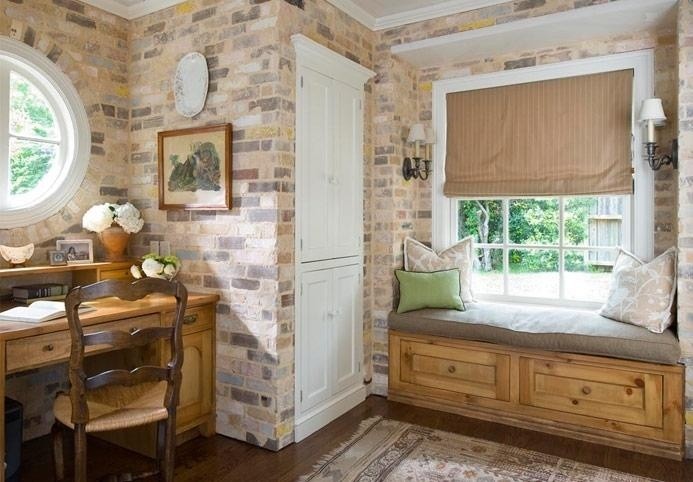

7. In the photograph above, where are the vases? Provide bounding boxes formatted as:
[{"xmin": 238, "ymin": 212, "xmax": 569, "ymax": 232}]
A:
[{"xmin": 99, "ymin": 223, "xmax": 129, "ymax": 262}]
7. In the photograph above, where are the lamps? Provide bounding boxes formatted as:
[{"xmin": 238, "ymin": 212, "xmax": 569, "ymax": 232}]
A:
[
  {"xmin": 403, "ymin": 122, "xmax": 435, "ymax": 181},
  {"xmin": 637, "ymin": 98, "xmax": 679, "ymax": 171}
]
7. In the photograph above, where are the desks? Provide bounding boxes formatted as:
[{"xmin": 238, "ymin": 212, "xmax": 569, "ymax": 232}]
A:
[{"xmin": 0, "ymin": 261, "xmax": 220, "ymax": 482}]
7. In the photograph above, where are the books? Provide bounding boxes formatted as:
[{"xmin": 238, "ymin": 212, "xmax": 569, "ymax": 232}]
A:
[
  {"xmin": 0, "ymin": 301, "xmax": 97, "ymax": 324},
  {"xmin": 11, "ymin": 283, "xmax": 68, "ymax": 299},
  {"xmin": 13, "ymin": 295, "xmax": 67, "ymax": 304}
]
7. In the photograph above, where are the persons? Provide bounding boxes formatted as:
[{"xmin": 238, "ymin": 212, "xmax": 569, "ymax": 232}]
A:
[{"xmin": 66, "ymin": 247, "xmax": 78, "ymax": 261}]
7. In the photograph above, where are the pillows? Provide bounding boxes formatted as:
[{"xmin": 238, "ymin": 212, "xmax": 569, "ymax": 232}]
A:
[
  {"xmin": 598, "ymin": 246, "xmax": 679, "ymax": 334},
  {"xmin": 393, "ymin": 268, "xmax": 466, "ymax": 314},
  {"xmin": 404, "ymin": 236, "xmax": 473, "ymax": 302}
]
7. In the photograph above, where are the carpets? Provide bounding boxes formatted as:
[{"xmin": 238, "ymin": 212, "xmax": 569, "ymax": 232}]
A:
[{"xmin": 296, "ymin": 413, "xmax": 662, "ymax": 482}]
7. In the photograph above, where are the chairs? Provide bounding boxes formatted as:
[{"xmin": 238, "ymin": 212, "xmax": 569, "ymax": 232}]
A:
[{"xmin": 49, "ymin": 277, "xmax": 189, "ymax": 482}]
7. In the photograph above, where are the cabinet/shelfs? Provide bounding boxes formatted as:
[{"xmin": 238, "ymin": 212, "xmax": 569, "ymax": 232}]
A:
[{"xmin": 386, "ymin": 296, "xmax": 683, "ymax": 461}]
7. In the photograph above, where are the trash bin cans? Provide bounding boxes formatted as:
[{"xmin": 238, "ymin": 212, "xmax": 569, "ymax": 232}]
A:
[{"xmin": 4, "ymin": 396, "xmax": 23, "ymax": 481}]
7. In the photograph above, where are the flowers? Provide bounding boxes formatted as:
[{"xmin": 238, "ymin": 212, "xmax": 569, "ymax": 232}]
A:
[
  {"xmin": 82, "ymin": 202, "xmax": 144, "ymax": 234},
  {"xmin": 131, "ymin": 253, "xmax": 181, "ymax": 282}
]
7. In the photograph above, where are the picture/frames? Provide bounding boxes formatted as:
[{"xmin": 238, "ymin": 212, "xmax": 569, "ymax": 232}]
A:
[
  {"xmin": 158, "ymin": 123, "xmax": 230, "ymax": 211},
  {"xmin": 50, "ymin": 250, "xmax": 68, "ymax": 266},
  {"xmin": 57, "ymin": 239, "xmax": 94, "ymax": 264}
]
